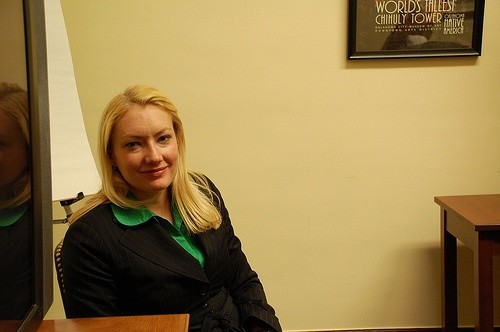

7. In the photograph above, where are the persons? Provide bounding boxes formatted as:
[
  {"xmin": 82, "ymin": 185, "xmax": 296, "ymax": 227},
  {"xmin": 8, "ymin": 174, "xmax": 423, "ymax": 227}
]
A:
[
  {"xmin": 61, "ymin": 85, "xmax": 282, "ymax": 332},
  {"xmin": 0, "ymin": 81, "xmax": 35, "ymax": 316}
]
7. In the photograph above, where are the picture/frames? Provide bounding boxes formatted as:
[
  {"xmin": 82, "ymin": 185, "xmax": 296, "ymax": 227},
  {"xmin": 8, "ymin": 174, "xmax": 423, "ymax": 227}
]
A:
[{"xmin": 346, "ymin": 0, "xmax": 486, "ymax": 61}]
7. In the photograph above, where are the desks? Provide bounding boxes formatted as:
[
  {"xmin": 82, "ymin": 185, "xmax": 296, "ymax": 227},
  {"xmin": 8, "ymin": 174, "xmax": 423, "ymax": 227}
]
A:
[
  {"xmin": 435, "ymin": 194, "xmax": 500, "ymax": 332},
  {"xmin": 37, "ymin": 313, "xmax": 191, "ymax": 332}
]
